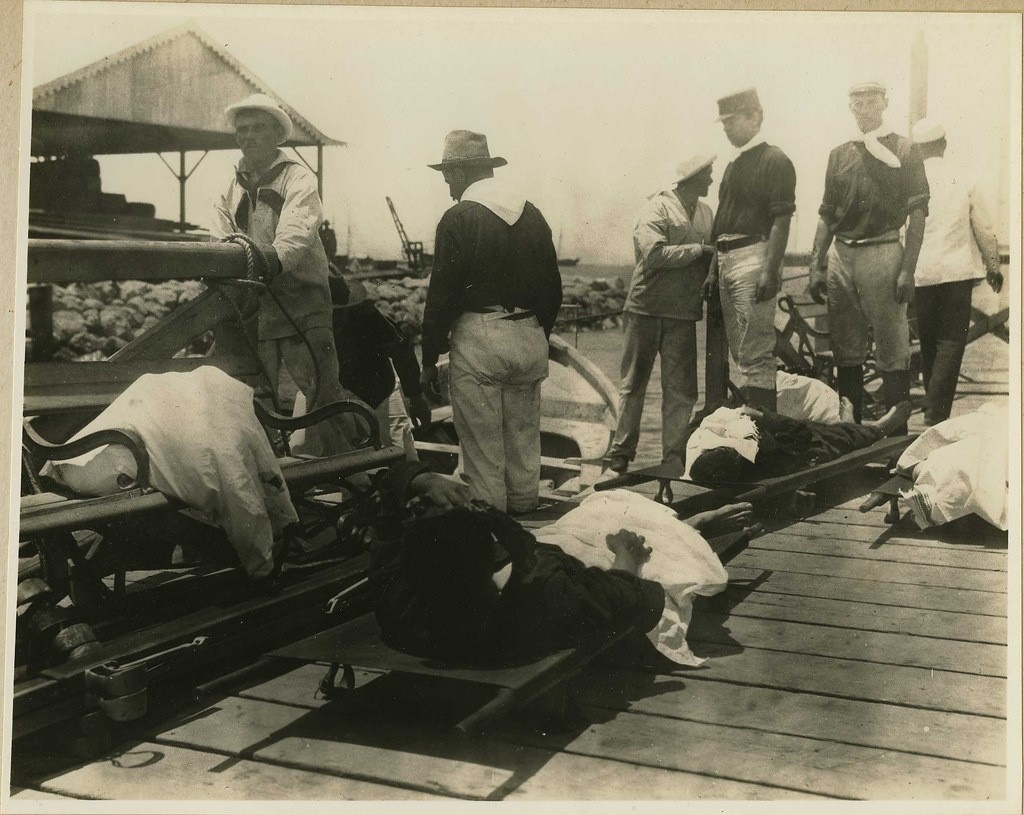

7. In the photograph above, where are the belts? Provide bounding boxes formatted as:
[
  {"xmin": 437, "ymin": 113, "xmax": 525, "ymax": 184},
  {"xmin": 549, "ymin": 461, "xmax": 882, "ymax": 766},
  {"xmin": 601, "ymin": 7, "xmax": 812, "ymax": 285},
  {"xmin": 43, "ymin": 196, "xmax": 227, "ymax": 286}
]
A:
[
  {"xmin": 834, "ymin": 238, "xmax": 900, "ymax": 247},
  {"xmin": 716, "ymin": 234, "xmax": 762, "ymax": 253},
  {"xmin": 465, "ymin": 307, "xmax": 536, "ymax": 320}
]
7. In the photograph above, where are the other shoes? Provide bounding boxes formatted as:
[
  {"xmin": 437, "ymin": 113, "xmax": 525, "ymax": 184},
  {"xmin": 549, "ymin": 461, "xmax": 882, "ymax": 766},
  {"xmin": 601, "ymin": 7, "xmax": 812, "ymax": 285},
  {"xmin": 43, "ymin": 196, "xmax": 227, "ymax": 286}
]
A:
[{"xmin": 609, "ymin": 457, "xmax": 629, "ymax": 472}]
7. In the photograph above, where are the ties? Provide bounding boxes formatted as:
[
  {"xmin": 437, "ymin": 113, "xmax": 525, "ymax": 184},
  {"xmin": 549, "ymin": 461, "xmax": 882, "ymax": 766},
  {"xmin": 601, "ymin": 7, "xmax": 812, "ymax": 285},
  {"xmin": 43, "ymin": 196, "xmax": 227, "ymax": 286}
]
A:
[
  {"xmin": 460, "ymin": 178, "xmax": 527, "ymax": 226},
  {"xmin": 237, "ymin": 161, "xmax": 287, "ymax": 212},
  {"xmin": 729, "ymin": 132, "xmax": 781, "ymax": 164},
  {"xmin": 838, "ymin": 123, "xmax": 901, "ymax": 168}
]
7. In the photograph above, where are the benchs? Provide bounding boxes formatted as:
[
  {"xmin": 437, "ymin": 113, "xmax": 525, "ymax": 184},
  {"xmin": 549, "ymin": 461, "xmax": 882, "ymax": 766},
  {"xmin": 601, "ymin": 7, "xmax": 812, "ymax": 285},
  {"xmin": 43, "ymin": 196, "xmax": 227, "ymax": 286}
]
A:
[
  {"xmin": 19, "ymin": 358, "xmax": 405, "ymax": 623},
  {"xmin": 775, "ymin": 290, "xmax": 923, "ymax": 414}
]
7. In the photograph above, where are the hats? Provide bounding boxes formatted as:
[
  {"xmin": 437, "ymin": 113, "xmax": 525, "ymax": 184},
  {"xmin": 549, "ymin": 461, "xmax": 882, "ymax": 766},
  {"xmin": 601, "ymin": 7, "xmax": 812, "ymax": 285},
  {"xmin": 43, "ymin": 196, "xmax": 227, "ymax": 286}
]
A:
[
  {"xmin": 670, "ymin": 144, "xmax": 717, "ymax": 185},
  {"xmin": 427, "ymin": 129, "xmax": 507, "ymax": 171},
  {"xmin": 714, "ymin": 86, "xmax": 761, "ymax": 123},
  {"xmin": 848, "ymin": 72, "xmax": 886, "ymax": 96},
  {"xmin": 911, "ymin": 118, "xmax": 944, "ymax": 144},
  {"xmin": 225, "ymin": 94, "xmax": 293, "ymax": 145}
]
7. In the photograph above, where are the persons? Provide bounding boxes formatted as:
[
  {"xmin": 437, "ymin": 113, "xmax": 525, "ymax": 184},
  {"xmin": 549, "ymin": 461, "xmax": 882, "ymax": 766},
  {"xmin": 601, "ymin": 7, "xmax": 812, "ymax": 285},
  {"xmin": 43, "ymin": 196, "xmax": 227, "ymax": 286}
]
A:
[
  {"xmin": 420, "ymin": 130, "xmax": 564, "ymax": 513},
  {"xmin": 677, "ymin": 402, "xmax": 912, "ymax": 486},
  {"xmin": 211, "ymin": 95, "xmax": 381, "ymax": 513},
  {"xmin": 810, "ymin": 75, "xmax": 930, "ymax": 434},
  {"xmin": 292, "ymin": 262, "xmax": 431, "ymax": 461},
  {"xmin": 900, "ymin": 118, "xmax": 1004, "ymax": 425},
  {"xmin": 608, "ymin": 153, "xmax": 715, "ymax": 479},
  {"xmin": 378, "ymin": 461, "xmax": 664, "ymax": 658},
  {"xmin": 703, "ymin": 88, "xmax": 796, "ymax": 413}
]
